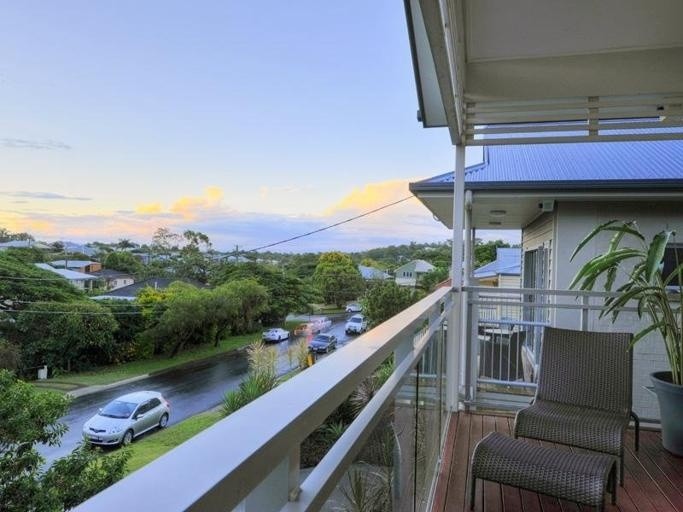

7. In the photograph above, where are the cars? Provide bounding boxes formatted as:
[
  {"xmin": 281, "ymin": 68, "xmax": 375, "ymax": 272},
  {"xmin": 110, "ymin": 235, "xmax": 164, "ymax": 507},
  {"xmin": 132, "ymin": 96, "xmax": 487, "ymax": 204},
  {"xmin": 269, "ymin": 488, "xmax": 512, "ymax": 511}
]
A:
[
  {"xmin": 293, "ymin": 322, "xmax": 319, "ymax": 338},
  {"xmin": 261, "ymin": 327, "xmax": 291, "ymax": 342},
  {"xmin": 344, "ymin": 302, "xmax": 362, "ymax": 313},
  {"xmin": 311, "ymin": 316, "xmax": 332, "ymax": 331},
  {"xmin": 79, "ymin": 389, "xmax": 171, "ymax": 450},
  {"xmin": 306, "ymin": 333, "xmax": 338, "ymax": 356}
]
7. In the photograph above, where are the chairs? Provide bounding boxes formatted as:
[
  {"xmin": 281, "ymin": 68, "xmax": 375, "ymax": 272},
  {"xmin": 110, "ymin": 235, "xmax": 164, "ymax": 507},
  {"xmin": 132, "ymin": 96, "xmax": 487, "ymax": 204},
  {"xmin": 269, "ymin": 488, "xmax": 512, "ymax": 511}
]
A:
[{"xmin": 466, "ymin": 329, "xmax": 637, "ymax": 511}]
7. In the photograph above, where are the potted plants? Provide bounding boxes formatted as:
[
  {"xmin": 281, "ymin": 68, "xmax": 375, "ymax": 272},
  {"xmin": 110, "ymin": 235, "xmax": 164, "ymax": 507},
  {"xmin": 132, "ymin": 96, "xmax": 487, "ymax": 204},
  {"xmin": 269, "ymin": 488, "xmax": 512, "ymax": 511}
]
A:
[{"xmin": 566, "ymin": 217, "xmax": 683, "ymax": 458}]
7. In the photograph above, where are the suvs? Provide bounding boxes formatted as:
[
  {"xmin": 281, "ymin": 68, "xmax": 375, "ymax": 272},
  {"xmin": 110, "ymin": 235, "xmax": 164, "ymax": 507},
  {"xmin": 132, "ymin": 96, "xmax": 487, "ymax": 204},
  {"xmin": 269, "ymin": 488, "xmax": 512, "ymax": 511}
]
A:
[{"xmin": 343, "ymin": 314, "xmax": 367, "ymax": 336}]
